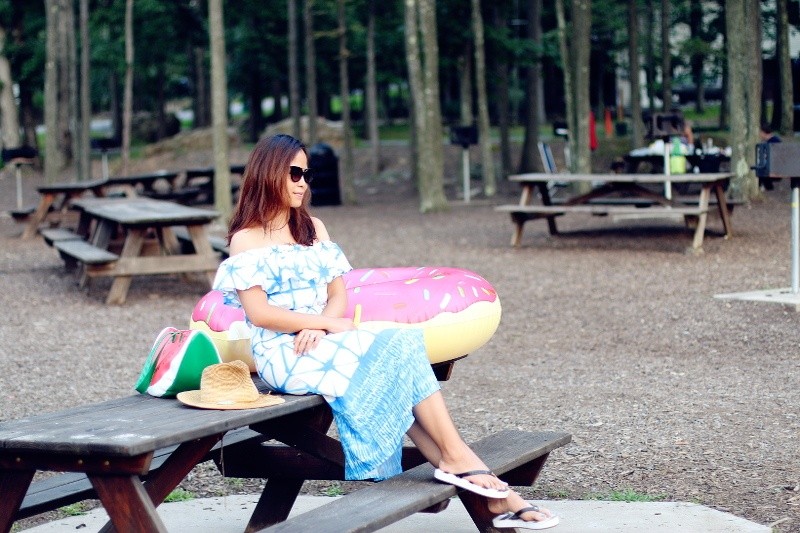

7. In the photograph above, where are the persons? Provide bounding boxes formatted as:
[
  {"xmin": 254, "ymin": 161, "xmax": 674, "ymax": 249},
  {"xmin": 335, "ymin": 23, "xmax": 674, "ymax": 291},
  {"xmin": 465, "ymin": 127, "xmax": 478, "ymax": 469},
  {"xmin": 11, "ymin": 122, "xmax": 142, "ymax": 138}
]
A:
[
  {"xmin": 212, "ymin": 134, "xmax": 561, "ymax": 530},
  {"xmin": 665, "ymin": 108, "xmax": 782, "ymax": 228}
]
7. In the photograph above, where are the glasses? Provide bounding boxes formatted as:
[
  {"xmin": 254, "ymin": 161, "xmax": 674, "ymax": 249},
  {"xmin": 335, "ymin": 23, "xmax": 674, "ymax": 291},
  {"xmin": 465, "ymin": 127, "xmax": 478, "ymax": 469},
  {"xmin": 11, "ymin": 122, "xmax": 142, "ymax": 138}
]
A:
[{"xmin": 289, "ymin": 166, "xmax": 314, "ymax": 184}]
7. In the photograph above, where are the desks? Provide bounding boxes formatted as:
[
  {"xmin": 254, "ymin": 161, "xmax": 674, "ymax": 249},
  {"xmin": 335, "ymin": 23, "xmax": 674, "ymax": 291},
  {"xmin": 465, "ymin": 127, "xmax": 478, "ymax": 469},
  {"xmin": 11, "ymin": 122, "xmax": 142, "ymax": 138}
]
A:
[
  {"xmin": 626, "ymin": 151, "xmax": 729, "ymax": 190},
  {"xmin": 113, "ymin": 171, "xmax": 181, "ymax": 198},
  {"xmin": 25, "ymin": 176, "xmax": 109, "ymax": 240},
  {"xmin": 509, "ymin": 172, "xmax": 733, "ymax": 249},
  {"xmin": 181, "ymin": 164, "xmax": 251, "ymax": 204},
  {"xmin": 67, "ymin": 196, "xmax": 220, "ymax": 307},
  {"xmin": 1, "ymin": 354, "xmax": 466, "ymax": 533}
]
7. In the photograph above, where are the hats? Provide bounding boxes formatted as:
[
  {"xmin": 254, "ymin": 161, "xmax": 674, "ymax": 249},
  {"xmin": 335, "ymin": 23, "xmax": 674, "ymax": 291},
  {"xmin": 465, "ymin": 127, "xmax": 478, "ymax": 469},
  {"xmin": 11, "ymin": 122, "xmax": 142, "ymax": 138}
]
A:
[{"xmin": 176, "ymin": 359, "xmax": 284, "ymax": 410}]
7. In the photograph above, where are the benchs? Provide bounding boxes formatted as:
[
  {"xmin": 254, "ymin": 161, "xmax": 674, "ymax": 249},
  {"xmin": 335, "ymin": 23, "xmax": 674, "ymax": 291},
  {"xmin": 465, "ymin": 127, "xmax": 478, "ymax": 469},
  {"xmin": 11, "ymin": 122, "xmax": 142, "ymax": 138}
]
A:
[
  {"xmin": 502, "ymin": 195, "xmax": 746, "ymax": 252},
  {"xmin": 1, "ymin": 428, "xmax": 572, "ymax": 533},
  {"xmin": 11, "ymin": 184, "xmax": 242, "ymax": 307}
]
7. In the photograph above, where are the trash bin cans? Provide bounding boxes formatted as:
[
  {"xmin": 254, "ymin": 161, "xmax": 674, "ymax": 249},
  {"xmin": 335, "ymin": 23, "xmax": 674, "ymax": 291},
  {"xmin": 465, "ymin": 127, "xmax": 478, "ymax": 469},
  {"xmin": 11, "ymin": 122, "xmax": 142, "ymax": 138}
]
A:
[{"xmin": 308, "ymin": 143, "xmax": 342, "ymax": 206}]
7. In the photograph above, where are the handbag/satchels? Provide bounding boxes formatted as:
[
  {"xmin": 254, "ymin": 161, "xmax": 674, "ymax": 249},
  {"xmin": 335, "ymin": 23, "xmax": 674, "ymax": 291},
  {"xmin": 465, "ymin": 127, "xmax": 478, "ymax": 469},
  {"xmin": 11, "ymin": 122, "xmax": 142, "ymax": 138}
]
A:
[{"xmin": 135, "ymin": 326, "xmax": 223, "ymax": 398}]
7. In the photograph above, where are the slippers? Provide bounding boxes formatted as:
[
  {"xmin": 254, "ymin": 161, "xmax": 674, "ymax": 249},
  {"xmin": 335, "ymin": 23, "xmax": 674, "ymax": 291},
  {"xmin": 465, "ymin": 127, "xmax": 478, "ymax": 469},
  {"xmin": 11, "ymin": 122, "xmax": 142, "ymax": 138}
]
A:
[
  {"xmin": 434, "ymin": 469, "xmax": 509, "ymax": 499},
  {"xmin": 493, "ymin": 503, "xmax": 559, "ymax": 530}
]
